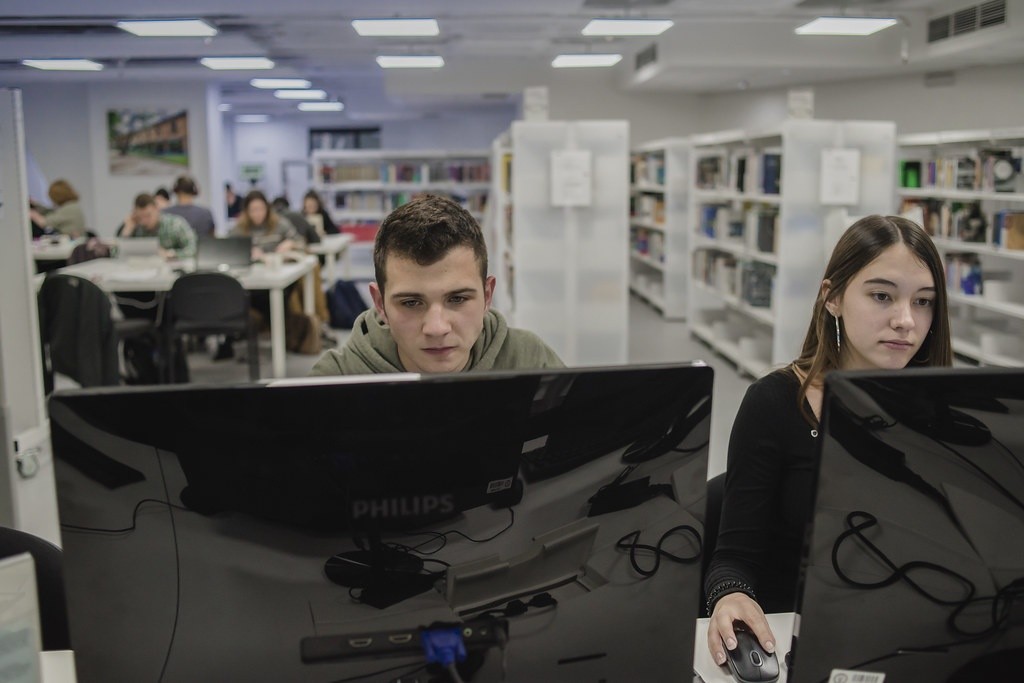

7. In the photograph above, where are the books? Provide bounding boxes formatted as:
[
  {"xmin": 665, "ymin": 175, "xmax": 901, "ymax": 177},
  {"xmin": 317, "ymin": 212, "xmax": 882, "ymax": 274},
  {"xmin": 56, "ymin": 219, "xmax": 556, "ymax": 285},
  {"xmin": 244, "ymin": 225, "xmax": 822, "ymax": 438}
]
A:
[
  {"xmin": 629, "ymin": 152, "xmax": 783, "ymax": 307},
  {"xmin": 321, "ymin": 156, "xmax": 521, "ymax": 297},
  {"xmin": 899, "ymin": 146, "xmax": 1024, "ymax": 294}
]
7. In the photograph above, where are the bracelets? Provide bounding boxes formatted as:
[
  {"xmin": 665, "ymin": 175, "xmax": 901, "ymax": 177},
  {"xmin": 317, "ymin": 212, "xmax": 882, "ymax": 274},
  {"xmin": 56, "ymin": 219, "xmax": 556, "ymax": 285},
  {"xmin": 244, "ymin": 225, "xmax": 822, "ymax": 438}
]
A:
[{"xmin": 706, "ymin": 580, "xmax": 757, "ymax": 616}]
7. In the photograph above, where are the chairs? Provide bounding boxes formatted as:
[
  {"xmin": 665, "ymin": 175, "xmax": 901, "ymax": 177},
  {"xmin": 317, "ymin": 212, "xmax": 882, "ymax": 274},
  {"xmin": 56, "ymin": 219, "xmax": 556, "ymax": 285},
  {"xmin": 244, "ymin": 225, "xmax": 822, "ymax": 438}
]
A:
[
  {"xmin": 0, "ymin": 527, "xmax": 72, "ymax": 650},
  {"xmin": 42, "ymin": 266, "xmax": 260, "ymax": 382}
]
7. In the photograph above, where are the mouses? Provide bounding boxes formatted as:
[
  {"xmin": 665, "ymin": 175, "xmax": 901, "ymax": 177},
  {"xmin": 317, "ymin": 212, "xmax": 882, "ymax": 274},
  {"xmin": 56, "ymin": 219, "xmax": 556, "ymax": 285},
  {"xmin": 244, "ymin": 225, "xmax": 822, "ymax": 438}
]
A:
[{"xmin": 722, "ymin": 630, "xmax": 780, "ymax": 683}]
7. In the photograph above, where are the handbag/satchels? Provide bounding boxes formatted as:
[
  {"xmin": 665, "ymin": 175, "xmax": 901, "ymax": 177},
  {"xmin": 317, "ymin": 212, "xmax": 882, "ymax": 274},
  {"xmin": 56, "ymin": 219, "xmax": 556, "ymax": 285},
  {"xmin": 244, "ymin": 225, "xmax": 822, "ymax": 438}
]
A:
[{"xmin": 285, "ymin": 314, "xmax": 320, "ymax": 353}]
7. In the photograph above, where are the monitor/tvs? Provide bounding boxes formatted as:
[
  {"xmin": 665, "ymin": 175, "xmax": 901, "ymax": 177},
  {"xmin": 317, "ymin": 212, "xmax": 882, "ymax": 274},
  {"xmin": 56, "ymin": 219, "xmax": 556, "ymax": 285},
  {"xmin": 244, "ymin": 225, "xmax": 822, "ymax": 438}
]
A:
[
  {"xmin": 789, "ymin": 365, "xmax": 1024, "ymax": 683},
  {"xmin": 48, "ymin": 357, "xmax": 716, "ymax": 683}
]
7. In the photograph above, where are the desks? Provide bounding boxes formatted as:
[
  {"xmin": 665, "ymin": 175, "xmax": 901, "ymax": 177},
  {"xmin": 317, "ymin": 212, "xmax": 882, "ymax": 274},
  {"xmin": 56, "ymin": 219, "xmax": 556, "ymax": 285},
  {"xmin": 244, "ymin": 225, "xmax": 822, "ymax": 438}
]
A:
[
  {"xmin": 35, "ymin": 252, "xmax": 316, "ymax": 384},
  {"xmin": 42, "ymin": 611, "xmax": 796, "ymax": 683}
]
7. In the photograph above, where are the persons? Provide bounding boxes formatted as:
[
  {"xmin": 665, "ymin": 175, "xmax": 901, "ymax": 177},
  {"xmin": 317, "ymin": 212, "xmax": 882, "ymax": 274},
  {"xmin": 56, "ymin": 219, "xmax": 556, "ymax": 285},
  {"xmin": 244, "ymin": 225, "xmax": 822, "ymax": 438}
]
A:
[
  {"xmin": 303, "ymin": 193, "xmax": 570, "ymax": 377},
  {"xmin": 27, "ymin": 180, "xmax": 87, "ymax": 257},
  {"xmin": 113, "ymin": 175, "xmax": 340, "ymax": 343},
  {"xmin": 704, "ymin": 215, "xmax": 956, "ymax": 667}
]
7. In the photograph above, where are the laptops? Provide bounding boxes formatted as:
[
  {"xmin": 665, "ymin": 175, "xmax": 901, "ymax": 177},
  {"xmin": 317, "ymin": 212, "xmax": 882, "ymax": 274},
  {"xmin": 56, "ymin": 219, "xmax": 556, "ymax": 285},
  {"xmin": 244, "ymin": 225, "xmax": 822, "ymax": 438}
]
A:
[
  {"xmin": 117, "ymin": 236, "xmax": 160, "ymax": 262},
  {"xmin": 194, "ymin": 236, "xmax": 254, "ymax": 281}
]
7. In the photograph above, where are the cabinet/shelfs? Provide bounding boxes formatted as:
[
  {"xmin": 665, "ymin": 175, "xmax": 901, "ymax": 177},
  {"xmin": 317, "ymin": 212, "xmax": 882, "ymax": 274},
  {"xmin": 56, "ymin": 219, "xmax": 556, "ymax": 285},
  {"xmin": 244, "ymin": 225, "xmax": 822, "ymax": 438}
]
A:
[
  {"xmin": 902, "ymin": 127, "xmax": 1024, "ymax": 371},
  {"xmin": 486, "ymin": 117, "xmax": 632, "ymax": 366},
  {"xmin": 632, "ymin": 137, "xmax": 693, "ymax": 321},
  {"xmin": 195, "ymin": 225, "xmax": 358, "ymax": 278},
  {"xmin": 688, "ymin": 119, "xmax": 902, "ymax": 381},
  {"xmin": 313, "ymin": 148, "xmax": 486, "ymax": 270}
]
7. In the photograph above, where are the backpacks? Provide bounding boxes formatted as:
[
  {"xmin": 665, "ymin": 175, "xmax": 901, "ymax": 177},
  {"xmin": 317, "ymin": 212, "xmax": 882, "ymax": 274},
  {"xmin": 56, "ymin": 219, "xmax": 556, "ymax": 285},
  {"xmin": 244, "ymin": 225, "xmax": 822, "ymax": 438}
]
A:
[{"xmin": 326, "ymin": 279, "xmax": 367, "ymax": 329}]
